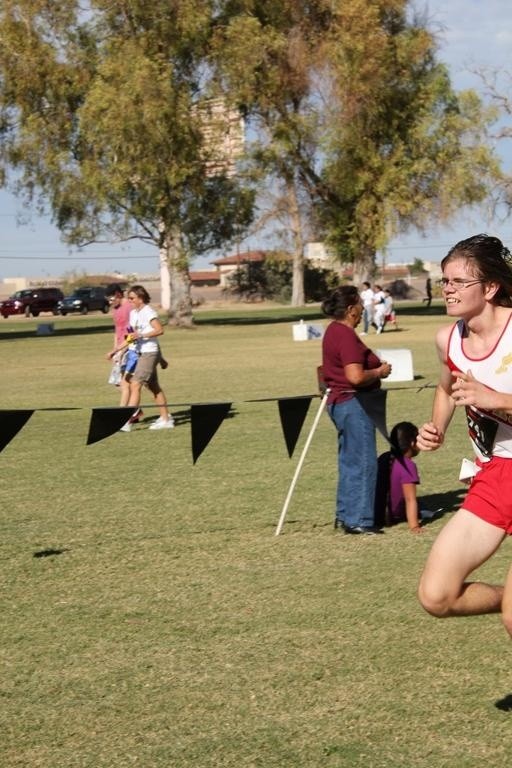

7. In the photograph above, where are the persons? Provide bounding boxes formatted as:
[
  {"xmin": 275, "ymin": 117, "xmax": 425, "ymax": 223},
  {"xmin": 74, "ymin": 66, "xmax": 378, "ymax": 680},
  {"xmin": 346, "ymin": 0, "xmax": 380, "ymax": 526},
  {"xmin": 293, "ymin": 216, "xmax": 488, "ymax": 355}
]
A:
[
  {"xmin": 422, "ymin": 278, "xmax": 432, "ymax": 307},
  {"xmin": 322, "ymin": 286, "xmax": 438, "ymax": 534},
  {"xmin": 103, "ymin": 283, "xmax": 175, "ymax": 432},
  {"xmin": 360, "ymin": 282, "xmax": 397, "ymax": 335},
  {"xmin": 416, "ymin": 234, "xmax": 512, "ymax": 639}
]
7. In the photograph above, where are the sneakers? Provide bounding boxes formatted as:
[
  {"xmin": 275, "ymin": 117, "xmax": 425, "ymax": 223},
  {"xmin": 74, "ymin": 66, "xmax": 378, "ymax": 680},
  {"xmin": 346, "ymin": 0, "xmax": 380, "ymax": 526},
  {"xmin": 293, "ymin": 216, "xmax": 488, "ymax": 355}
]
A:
[
  {"xmin": 148, "ymin": 419, "xmax": 173, "ymax": 430},
  {"xmin": 128, "ymin": 408, "xmax": 144, "ymax": 424},
  {"xmin": 121, "ymin": 422, "xmax": 134, "ymax": 433}
]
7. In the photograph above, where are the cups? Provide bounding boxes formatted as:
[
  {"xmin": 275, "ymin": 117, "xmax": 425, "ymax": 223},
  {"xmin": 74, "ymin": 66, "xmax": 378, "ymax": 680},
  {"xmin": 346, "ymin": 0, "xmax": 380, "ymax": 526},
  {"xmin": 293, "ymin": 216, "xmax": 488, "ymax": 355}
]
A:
[{"xmin": 126, "ymin": 324, "xmax": 138, "ymax": 345}]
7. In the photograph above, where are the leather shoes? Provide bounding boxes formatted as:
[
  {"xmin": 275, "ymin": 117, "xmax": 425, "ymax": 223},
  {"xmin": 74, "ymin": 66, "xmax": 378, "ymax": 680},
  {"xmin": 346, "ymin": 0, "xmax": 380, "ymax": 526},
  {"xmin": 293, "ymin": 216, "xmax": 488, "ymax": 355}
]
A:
[{"xmin": 334, "ymin": 518, "xmax": 385, "ymax": 535}]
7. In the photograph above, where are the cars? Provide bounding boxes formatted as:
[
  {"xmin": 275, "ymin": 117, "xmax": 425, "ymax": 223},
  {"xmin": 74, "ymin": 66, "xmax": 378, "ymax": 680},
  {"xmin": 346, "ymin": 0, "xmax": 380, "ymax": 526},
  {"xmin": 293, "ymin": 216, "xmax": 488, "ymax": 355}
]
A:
[{"xmin": 0, "ymin": 286, "xmax": 110, "ymax": 318}]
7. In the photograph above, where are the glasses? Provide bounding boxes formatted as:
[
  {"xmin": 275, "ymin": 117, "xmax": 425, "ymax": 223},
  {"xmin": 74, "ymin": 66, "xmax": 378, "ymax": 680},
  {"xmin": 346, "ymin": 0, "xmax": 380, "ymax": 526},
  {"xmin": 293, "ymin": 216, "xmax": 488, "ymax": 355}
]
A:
[
  {"xmin": 128, "ymin": 297, "xmax": 135, "ymax": 301},
  {"xmin": 436, "ymin": 278, "xmax": 489, "ymax": 290}
]
